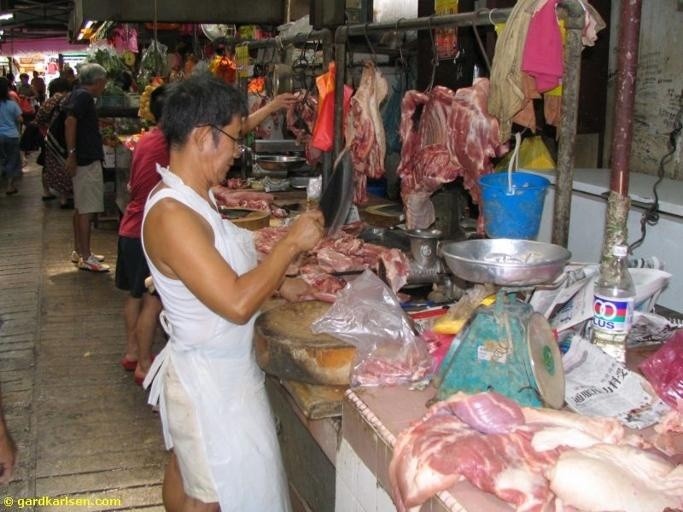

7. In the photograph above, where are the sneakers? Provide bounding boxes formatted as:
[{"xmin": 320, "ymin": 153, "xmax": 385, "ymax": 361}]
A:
[
  {"xmin": 42, "ymin": 194, "xmax": 56, "ymax": 200},
  {"xmin": 78, "ymin": 253, "xmax": 110, "ymax": 272},
  {"xmin": 6, "ymin": 188, "xmax": 18, "ymax": 195},
  {"xmin": 61, "ymin": 199, "xmax": 74, "ymax": 209},
  {"xmin": 72, "ymin": 250, "xmax": 105, "ymax": 262}
]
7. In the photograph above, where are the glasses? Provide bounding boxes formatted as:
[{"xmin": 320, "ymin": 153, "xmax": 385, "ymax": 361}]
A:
[{"xmin": 196, "ymin": 124, "xmax": 244, "ymax": 147}]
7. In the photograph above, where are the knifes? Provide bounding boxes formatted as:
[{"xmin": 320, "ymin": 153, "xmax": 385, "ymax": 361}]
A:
[{"xmin": 315, "ymin": 149, "xmax": 353, "ymax": 231}]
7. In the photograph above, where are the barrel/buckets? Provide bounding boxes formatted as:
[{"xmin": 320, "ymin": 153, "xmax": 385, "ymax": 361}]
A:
[{"xmin": 475, "ymin": 131, "xmax": 551, "ymax": 240}]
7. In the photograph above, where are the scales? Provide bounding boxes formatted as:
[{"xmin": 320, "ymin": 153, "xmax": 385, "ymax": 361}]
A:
[
  {"xmin": 425, "ymin": 237, "xmax": 571, "ymax": 409},
  {"xmin": 255, "ymin": 36, "xmax": 307, "ymax": 170}
]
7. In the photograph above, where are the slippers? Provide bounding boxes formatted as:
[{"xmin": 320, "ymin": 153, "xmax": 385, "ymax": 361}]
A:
[{"xmin": 122, "ymin": 352, "xmax": 158, "ymax": 370}]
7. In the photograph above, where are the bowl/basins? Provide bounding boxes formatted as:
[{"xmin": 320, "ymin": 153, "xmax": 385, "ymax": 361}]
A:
[
  {"xmin": 256, "ymin": 155, "xmax": 306, "ymax": 171},
  {"xmin": 201, "ymin": 22, "xmax": 236, "ymax": 44},
  {"xmin": 271, "ymin": 62, "xmax": 295, "ymax": 99}
]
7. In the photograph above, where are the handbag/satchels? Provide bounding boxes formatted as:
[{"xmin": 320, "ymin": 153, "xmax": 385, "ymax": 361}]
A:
[
  {"xmin": 19, "ymin": 126, "xmax": 40, "ymax": 151},
  {"xmin": 19, "ymin": 97, "xmax": 36, "ymax": 115},
  {"xmin": 45, "ymin": 112, "xmax": 76, "ymax": 164}
]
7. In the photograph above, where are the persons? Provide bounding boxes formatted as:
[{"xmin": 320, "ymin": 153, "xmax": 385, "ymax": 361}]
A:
[
  {"xmin": 0, "ymin": 393, "xmax": 17, "ymax": 485},
  {"xmin": 1, "ymin": 63, "xmax": 75, "ymax": 210},
  {"xmin": 142, "ymin": 76, "xmax": 325, "ymax": 511},
  {"xmin": 63, "ymin": 62, "xmax": 111, "ymax": 273},
  {"xmin": 115, "ymin": 83, "xmax": 297, "ymax": 388}
]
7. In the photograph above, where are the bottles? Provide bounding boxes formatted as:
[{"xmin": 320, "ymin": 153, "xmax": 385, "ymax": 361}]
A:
[{"xmin": 592, "ymin": 245, "xmax": 635, "ymax": 364}]
